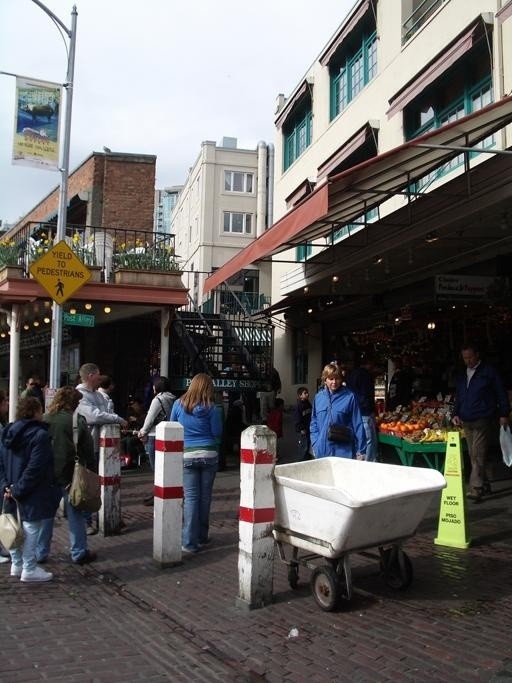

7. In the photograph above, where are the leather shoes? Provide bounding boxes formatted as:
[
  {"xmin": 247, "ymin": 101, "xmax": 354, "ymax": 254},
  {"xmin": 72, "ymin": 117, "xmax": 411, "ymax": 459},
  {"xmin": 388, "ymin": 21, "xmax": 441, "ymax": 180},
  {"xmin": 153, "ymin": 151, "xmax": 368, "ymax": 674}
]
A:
[{"xmin": 72, "ymin": 547, "xmax": 98, "ymax": 564}]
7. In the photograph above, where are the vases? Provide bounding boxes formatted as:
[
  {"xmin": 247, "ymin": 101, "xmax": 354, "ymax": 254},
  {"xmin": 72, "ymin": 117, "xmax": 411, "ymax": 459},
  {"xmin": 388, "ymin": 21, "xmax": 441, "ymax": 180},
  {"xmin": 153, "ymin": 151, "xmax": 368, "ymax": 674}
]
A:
[
  {"xmin": 85, "ymin": 263, "xmax": 104, "ymax": 282},
  {"xmin": 0, "ymin": 264, "xmax": 24, "ymax": 283},
  {"xmin": 114, "ymin": 269, "xmax": 184, "ymax": 290}
]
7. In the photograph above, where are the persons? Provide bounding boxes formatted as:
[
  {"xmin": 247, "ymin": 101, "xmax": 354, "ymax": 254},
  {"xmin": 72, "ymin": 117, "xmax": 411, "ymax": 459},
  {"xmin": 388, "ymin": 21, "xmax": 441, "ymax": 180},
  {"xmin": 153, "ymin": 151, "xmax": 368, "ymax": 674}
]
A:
[
  {"xmin": 308, "ymin": 360, "xmax": 368, "ymax": 462},
  {"xmin": 18, "ymin": 376, "xmax": 47, "ymax": 415},
  {"xmin": 170, "ymin": 372, "xmax": 225, "ymax": 555},
  {"xmin": 121, "ymin": 397, "xmax": 145, "ymax": 471},
  {"xmin": 268, "ymin": 398, "xmax": 285, "ymax": 462},
  {"xmin": 450, "ymin": 344, "xmax": 509, "ymax": 501},
  {"xmin": 221, "ymin": 398, "xmax": 248, "ymax": 472},
  {"xmin": 98, "ymin": 374, "xmax": 116, "ymax": 415},
  {"xmin": 295, "ymin": 386, "xmax": 313, "ymax": 461},
  {"xmin": 75, "ymin": 363, "xmax": 128, "ymax": 536},
  {"xmin": 0, "ymin": 389, "xmax": 10, "ymax": 425},
  {"xmin": 36, "ymin": 384, "xmax": 99, "ymax": 567},
  {"xmin": 137, "ymin": 374, "xmax": 179, "ymax": 507},
  {"xmin": 0, "ymin": 397, "xmax": 55, "ymax": 582}
]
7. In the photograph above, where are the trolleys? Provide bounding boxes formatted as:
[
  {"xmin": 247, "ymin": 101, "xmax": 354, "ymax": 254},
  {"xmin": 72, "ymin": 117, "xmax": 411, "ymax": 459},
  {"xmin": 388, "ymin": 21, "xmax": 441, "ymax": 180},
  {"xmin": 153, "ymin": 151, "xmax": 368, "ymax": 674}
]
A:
[{"xmin": 273, "ymin": 457, "xmax": 447, "ymax": 610}]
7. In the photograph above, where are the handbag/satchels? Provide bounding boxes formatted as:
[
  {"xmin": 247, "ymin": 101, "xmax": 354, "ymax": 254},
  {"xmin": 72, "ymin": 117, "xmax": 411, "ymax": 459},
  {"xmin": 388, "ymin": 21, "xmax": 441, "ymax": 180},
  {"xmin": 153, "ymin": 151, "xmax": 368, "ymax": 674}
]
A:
[
  {"xmin": 68, "ymin": 412, "xmax": 103, "ymax": 516},
  {"xmin": 0, "ymin": 493, "xmax": 25, "ymax": 550},
  {"xmin": 152, "ymin": 397, "xmax": 168, "ymax": 426},
  {"xmin": 327, "ymin": 392, "xmax": 354, "ymax": 446}
]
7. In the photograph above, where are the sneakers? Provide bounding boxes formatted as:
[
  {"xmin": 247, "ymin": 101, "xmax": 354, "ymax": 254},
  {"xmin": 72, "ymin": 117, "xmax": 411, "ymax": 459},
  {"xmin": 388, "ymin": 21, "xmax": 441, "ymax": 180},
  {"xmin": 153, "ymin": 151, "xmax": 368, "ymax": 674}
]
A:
[
  {"xmin": 181, "ymin": 544, "xmax": 198, "ymax": 554},
  {"xmin": 11, "ymin": 562, "xmax": 23, "ymax": 577},
  {"xmin": 197, "ymin": 538, "xmax": 213, "ymax": 548},
  {"xmin": 465, "ymin": 487, "xmax": 482, "ymax": 499},
  {"xmin": 20, "ymin": 567, "xmax": 54, "ymax": 582}
]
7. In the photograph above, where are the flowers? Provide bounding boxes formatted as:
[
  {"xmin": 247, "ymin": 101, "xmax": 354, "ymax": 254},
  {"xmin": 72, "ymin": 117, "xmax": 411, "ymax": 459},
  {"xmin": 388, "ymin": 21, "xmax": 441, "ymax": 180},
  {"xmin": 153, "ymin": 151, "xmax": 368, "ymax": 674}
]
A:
[
  {"xmin": 113, "ymin": 238, "xmax": 179, "ymax": 272},
  {"xmin": 30, "ymin": 229, "xmax": 97, "ymax": 266},
  {"xmin": 0, "ymin": 236, "xmax": 21, "ymax": 269}
]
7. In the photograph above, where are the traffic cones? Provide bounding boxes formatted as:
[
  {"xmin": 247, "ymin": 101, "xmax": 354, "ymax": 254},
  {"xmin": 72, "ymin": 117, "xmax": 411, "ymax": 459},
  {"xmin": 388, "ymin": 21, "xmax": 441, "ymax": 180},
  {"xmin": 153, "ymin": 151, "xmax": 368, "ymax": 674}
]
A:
[{"xmin": 433, "ymin": 432, "xmax": 467, "ymax": 547}]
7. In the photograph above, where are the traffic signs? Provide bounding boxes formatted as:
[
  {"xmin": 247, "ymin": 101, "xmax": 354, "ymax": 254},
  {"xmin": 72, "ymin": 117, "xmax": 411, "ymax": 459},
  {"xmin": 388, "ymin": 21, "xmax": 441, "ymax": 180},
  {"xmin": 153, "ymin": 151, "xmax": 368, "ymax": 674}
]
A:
[{"xmin": 28, "ymin": 242, "xmax": 92, "ymax": 303}]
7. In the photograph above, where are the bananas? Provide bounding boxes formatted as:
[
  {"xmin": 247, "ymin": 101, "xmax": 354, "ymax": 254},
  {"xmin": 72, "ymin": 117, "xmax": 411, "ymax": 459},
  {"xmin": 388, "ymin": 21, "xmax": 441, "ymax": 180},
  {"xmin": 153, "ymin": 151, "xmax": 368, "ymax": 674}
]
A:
[{"xmin": 419, "ymin": 429, "xmax": 448, "ymax": 442}]
